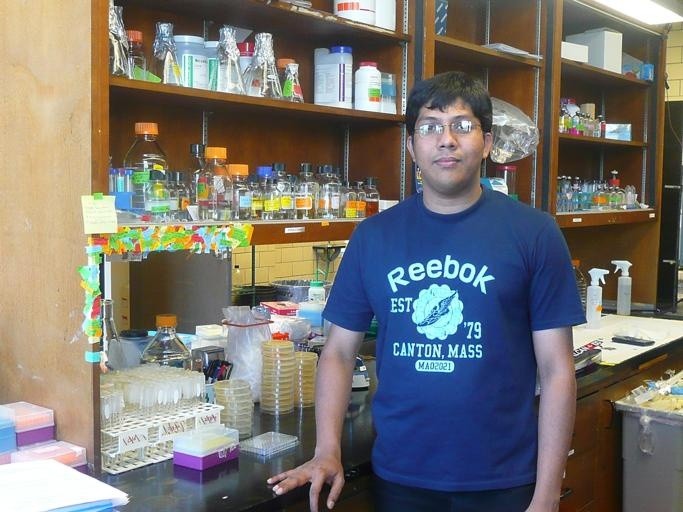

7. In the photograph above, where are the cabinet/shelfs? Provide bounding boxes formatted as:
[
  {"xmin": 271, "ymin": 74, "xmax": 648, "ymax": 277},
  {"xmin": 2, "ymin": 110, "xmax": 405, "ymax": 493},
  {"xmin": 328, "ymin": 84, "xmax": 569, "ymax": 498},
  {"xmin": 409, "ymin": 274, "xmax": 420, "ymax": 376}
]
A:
[
  {"xmin": 89, "ymin": 0, "xmax": 417, "ymax": 246},
  {"xmin": 547, "ymin": 0, "xmax": 664, "ymax": 228},
  {"xmin": 534, "ymin": 332, "xmax": 682, "ymax": 512},
  {"xmin": 419, "ymin": 1, "xmax": 553, "ymax": 220}
]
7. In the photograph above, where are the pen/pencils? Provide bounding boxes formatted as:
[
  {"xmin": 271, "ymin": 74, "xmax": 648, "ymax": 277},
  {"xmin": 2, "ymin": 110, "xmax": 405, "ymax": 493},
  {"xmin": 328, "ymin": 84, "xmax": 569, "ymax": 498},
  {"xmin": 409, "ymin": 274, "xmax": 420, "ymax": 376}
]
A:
[{"xmin": 204, "ymin": 359, "xmax": 233, "ymax": 383}]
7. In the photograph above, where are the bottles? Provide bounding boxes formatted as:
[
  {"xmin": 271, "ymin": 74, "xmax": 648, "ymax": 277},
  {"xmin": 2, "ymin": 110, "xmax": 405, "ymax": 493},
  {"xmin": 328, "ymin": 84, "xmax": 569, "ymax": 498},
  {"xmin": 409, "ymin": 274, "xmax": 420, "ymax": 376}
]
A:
[
  {"xmin": 568, "ymin": 259, "xmax": 585, "ymax": 310},
  {"xmin": 98, "ymin": 364, "xmax": 206, "ymax": 470},
  {"xmin": 106, "ymin": 2, "xmax": 397, "ymax": 115},
  {"xmin": 556, "ymin": 98, "xmax": 605, "ymax": 138},
  {"xmin": 141, "ymin": 312, "xmax": 189, "ymax": 369},
  {"xmin": 494, "ymin": 165, "xmax": 520, "ymax": 198},
  {"xmin": 231, "ymin": 265, "xmax": 243, "ymax": 307},
  {"xmin": 185, "ymin": 358, "xmax": 203, "ymax": 373},
  {"xmin": 306, "ymin": 280, "xmax": 326, "ymax": 303},
  {"xmin": 99, "ymin": 298, "xmax": 128, "ymax": 371},
  {"xmin": 553, "ymin": 176, "xmax": 638, "ymax": 212},
  {"xmin": 109, "ymin": 120, "xmax": 379, "ymax": 224}
]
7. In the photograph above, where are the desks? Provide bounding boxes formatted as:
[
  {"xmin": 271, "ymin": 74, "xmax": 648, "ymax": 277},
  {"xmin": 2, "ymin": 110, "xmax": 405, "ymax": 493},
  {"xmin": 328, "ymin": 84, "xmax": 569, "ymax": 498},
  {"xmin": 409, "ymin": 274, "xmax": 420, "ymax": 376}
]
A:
[{"xmin": 102, "ymin": 357, "xmax": 379, "ymax": 512}]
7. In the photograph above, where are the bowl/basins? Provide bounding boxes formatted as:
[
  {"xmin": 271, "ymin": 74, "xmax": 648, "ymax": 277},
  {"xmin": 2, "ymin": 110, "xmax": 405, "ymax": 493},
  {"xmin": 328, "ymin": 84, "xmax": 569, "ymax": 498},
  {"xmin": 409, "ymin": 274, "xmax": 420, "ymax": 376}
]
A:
[{"xmin": 271, "ymin": 280, "xmax": 334, "ymax": 302}]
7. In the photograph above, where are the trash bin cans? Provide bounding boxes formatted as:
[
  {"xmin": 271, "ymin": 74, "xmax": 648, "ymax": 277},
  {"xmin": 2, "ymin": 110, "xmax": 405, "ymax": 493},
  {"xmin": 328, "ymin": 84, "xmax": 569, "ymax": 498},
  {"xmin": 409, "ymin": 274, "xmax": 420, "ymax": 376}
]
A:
[{"xmin": 615, "ymin": 369, "xmax": 683, "ymax": 512}]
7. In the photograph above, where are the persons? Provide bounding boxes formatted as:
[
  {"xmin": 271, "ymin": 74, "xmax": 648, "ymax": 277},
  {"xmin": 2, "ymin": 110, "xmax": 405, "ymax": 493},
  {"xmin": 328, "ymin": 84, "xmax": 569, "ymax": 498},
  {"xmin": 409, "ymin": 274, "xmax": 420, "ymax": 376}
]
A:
[{"xmin": 263, "ymin": 70, "xmax": 588, "ymax": 511}]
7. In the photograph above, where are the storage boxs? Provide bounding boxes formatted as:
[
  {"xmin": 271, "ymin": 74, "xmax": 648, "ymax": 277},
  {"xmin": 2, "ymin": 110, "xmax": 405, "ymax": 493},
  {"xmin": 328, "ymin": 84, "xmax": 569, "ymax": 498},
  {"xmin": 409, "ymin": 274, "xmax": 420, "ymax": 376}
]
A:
[{"xmin": 562, "ymin": 22, "xmax": 623, "ymax": 74}]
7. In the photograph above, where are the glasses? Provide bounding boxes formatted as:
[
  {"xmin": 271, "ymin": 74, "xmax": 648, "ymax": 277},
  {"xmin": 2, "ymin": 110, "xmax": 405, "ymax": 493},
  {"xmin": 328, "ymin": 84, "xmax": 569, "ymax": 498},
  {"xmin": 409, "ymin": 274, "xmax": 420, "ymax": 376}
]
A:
[{"xmin": 414, "ymin": 120, "xmax": 483, "ymax": 139}]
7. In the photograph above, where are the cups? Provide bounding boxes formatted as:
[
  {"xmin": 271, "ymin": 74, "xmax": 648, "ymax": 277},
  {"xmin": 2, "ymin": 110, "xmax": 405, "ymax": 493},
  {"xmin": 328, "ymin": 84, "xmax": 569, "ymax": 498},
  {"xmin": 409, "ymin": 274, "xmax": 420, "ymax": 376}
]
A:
[
  {"xmin": 215, "ymin": 378, "xmax": 255, "ymax": 440},
  {"xmin": 203, "ymin": 384, "xmax": 216, "ymax": 404},
  {"xmin": 260, "ymin": 339, "xmax": 296, "ymax": 414},
  {"xmin": 291, "ymin": 351, "xmax": 318, "ymax": 409}
]
